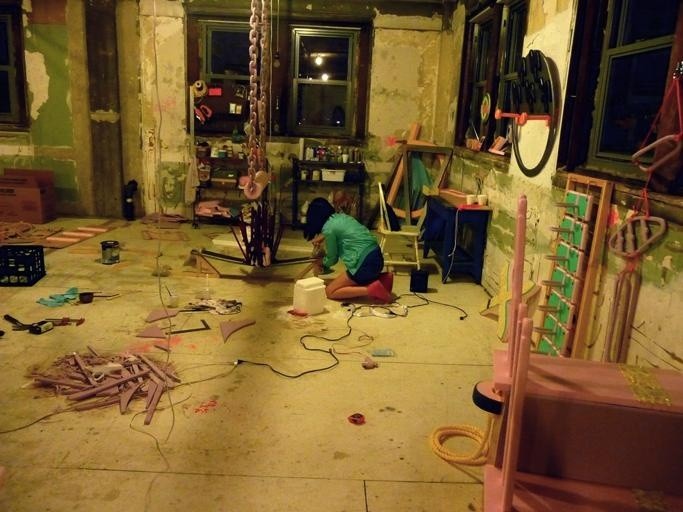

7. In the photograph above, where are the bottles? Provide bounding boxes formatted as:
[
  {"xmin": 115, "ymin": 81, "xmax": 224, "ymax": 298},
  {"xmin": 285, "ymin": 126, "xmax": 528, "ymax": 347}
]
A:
[
  {"xmin": 305, "ymin": 144, "xmax": 360, "ymax": 162},
  {"xmin": 195, "ymin": 138, "xmax": 234, "ymax": 161}
]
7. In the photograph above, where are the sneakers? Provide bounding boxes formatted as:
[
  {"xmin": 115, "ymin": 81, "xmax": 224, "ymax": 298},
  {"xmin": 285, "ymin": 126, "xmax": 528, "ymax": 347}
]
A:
[
  {"xmin": 367, "ymin": 279, "xmax": 392, "ymax": 304},
  {"xmin": 378, "ymin": 272, "xmax": 392, "ymax": 294}
]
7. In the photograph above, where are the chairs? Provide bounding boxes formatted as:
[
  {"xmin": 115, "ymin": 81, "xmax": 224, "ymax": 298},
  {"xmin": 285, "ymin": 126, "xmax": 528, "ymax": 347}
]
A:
[{"xmin": 376, "ymin": 183, "xmax": 420, "ymax": 273}]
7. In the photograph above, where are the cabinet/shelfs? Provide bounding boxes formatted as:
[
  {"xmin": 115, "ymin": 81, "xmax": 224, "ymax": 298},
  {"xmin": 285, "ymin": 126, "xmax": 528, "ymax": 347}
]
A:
[
  {"xmin": 190, "ymin": 139, "xmax": 259, "ymax": 230},
  {"xmin": 480, "ymin": 195, "xmax": 682, "ymax": 512}
]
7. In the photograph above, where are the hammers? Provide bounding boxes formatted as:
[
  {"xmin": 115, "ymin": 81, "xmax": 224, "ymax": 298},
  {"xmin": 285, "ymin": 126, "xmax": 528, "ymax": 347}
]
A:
[{"xmin": 4, "ymin": 314, "xmax": 32, "ymax": 330}]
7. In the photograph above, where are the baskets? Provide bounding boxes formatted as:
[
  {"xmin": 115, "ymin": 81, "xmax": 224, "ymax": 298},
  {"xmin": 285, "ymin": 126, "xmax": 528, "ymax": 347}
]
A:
[{"xmin": 0, "ymin": 245, "xmax": 46, "ymax": 287}]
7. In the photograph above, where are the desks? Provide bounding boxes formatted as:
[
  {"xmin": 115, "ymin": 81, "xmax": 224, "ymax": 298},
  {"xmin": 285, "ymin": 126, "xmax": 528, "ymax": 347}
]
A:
[
  {"xmin": 424, "ymin": 196, "xmax": 490, "ymax": 285},
  {"xmin": 288, "ymin": 151, "xmax": 364, "ymax": 224}
]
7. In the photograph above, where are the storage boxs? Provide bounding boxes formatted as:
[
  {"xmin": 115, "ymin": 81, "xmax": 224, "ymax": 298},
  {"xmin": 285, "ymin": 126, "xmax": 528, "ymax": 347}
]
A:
[{"xmin": 0, "ymin": 168, "xmax": 56, "ymax": 226}]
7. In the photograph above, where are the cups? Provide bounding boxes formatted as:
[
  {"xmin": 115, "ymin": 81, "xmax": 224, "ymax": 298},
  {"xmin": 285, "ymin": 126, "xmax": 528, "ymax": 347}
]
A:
[
  {"xmin": 477, "ymin": 195, "xmax": 487, "ymax": 205},
  {"xmin": 466, "ymin": 195, "xmax": 477, "ymax": 205},
  {"xmin": 78, "ymin": 292, "xmax": 93, "ymax": 303}
]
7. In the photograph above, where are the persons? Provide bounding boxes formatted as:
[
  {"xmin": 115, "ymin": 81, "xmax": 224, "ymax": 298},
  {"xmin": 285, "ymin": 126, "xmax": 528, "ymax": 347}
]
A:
[{"xmin": 302, "ymin": 197, "xmax": 396, "ymax": 305}]
